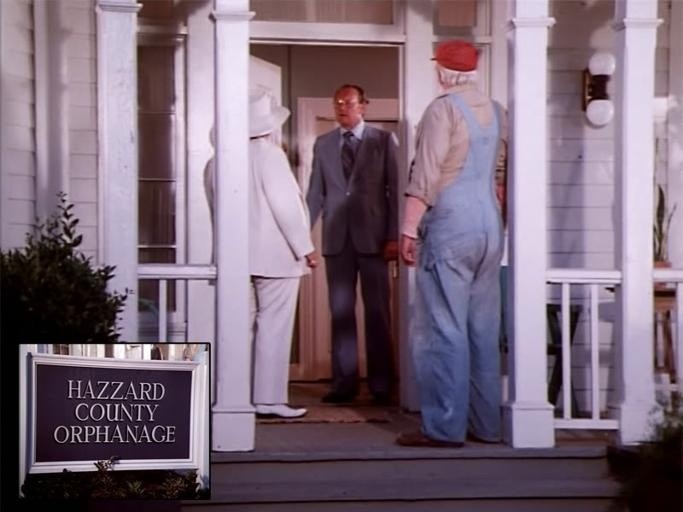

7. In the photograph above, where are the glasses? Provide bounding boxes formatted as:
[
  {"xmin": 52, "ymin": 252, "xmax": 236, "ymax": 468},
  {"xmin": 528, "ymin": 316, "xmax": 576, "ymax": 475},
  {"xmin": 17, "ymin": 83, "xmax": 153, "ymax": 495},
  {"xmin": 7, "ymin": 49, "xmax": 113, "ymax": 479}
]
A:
[{"xmin": 331, "ymin": 97, "xmax": 362, "ymax": 109}]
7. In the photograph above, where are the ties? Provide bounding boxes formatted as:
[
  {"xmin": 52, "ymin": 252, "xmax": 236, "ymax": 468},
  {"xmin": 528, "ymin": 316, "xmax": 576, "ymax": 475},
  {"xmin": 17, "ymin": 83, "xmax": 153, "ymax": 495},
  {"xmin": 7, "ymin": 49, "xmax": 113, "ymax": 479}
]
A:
[{"xmin": 342, "ymin": 132, "xmax": 356, "ymax": 180}]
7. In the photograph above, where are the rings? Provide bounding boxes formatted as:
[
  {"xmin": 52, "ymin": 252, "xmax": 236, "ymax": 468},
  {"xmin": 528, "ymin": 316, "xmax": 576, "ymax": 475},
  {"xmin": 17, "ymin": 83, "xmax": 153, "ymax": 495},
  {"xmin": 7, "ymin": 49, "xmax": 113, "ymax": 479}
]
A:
[{"xmin": 310, "ymin": 260, "xmax": 317, "ymax": 266}]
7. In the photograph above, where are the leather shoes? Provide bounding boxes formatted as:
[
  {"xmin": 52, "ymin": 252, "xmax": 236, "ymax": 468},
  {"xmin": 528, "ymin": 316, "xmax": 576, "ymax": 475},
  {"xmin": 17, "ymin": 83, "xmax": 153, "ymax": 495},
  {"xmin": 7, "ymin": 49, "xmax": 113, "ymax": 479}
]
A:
[
  {"xmin": 256, "ymin": 403, "xmax": 309, "ymax": 418},
  {"xmin": 375, "ymin": 393, "xmax": 392, "ymax": 406},
  {"xmin": 396, "ymin": 432, "xmax": 465, "ymax": 447},
  {"xmin": 320, "ymin": 391, "xmax": 357, "ymax": 403}
]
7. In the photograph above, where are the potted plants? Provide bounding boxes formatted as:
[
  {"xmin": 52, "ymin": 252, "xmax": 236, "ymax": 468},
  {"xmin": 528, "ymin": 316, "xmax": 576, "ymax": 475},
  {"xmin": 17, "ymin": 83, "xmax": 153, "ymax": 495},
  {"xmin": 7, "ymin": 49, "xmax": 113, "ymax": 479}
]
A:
[{"xmin": 652, "ymin": 186, "xmax": 678, "ymax": 292}]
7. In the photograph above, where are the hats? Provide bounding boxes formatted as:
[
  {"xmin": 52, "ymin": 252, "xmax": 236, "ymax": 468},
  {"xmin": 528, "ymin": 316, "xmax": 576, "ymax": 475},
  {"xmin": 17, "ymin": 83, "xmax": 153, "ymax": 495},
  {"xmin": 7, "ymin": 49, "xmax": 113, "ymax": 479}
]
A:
[
  {"xmin": 431, "ymin": 40, "xmax": 478, "ymax": 71},
  {"xmin": 249, "ymin": 87, "xmax": 292, "ymax": 139}
]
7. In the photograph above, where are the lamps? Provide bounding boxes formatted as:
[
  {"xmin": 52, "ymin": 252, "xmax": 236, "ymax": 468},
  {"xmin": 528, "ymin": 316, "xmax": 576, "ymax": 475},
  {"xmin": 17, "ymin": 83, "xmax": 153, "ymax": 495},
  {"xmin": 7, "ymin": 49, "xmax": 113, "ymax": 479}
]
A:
[{"xmin": 581, "ymin": 50, "xmax": 615, "ymax": 125}]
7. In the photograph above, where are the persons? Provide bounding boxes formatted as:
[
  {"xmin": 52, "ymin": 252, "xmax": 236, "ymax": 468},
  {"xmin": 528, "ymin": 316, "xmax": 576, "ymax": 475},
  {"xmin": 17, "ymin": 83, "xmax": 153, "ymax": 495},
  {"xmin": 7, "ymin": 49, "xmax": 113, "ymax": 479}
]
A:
[
  {"xmin": 306, "ymin": 85, "xmax": 397, "ymax": 406},
  {"xmin": 393, "ymin": 38, "xmax": 508, "ymax": 449},
  {"xmin": 204, "ymin": 92, "xmax": 319, "ymax": 420}
]
